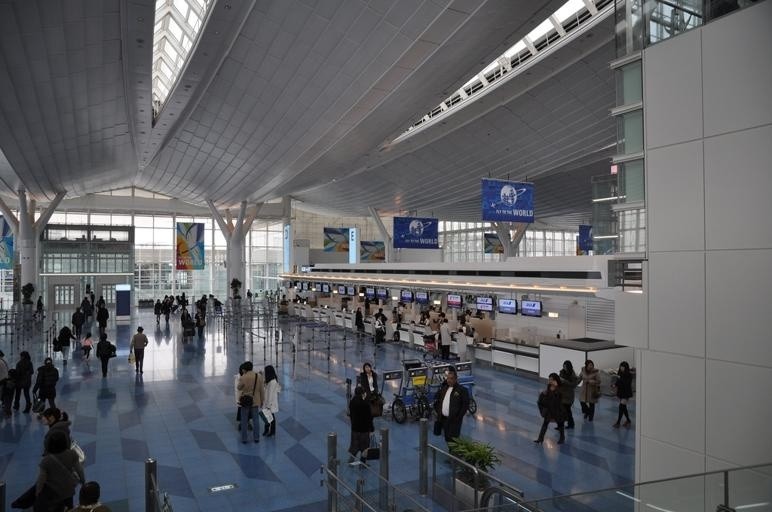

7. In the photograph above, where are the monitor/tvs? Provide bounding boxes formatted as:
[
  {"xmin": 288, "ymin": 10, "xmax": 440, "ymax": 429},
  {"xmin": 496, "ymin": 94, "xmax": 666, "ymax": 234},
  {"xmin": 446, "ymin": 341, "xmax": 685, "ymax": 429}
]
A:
[
  {"xmin": 499, "ymin": 298, "xmax": 517, "ymax": 314},
  {"xmin": 447, "ymin": 294, "xmax": 462, "ymax": 308},
  {"xmin": 476, "ymin": 297, "xmax": 493, "ymax": 311},
  {"xmin": 401, "ymin": 290, "xmax": 413, "ymax": 303},
  {"xmin": 285, "ymin": 281, "xmax": 387, "ymax": 299},
  {"xmin": 416, "ymin": 292, "xmax": 428, "ymax": 304},
  {"xmin": 521, "ymin": 300, "xmax": 542, "ymax": 317}
]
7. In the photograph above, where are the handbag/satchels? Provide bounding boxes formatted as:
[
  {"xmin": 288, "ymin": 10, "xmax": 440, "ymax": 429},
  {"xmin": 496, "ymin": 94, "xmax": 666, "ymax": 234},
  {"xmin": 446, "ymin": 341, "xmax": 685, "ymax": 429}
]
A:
[
  {"xmin": 69, "ymin": 441, "xmax": 86, "ymax": 464},
  {"xmin": 108, "ymin": 345, "xmax": 116, "ymax": 358},
  {"xmin": 537, "ymin": 390, "xmax": 549, "ymax": 418},
  {"xmin": 592, "ymin": 389, "xmax": 601, "ymax": 398},
  {"xmin": 259, "ymin": 406, "xmax": 274, "ymax": 425},
  {"xmin": 361, "ymin": 444, "xmax": 380, "ymax": 460},
  {"xmin": 610, "ymin": 384, "xmax": 618, "ymax": 396},
  {"xmin": 432, "ymin": 418, "xmax": 441, "ymax": 436},
  {"xmin": 71, "ymin": 326, "xmax": 75, "ymax": 335},
  {"xmin": 366, "ymin": 392, "xmax": 384, "ymax": 418},
  {"xmin": 128, "ymin": 352, "xmax": 136, "ymax": 366},
  {"xmin": 240, "ymin": 394, "xmax": 254, "ymax": 408},
  {"xmin": 53, "ymin": 339, "xmax": 62, "ymax": 352},
  {"xmin": 31, "ymin": 397, "xmax": 45, "ymax": 413},
  {"xmin": 8, "ymin": 368, "xmax": 17, "ymax": 380}
]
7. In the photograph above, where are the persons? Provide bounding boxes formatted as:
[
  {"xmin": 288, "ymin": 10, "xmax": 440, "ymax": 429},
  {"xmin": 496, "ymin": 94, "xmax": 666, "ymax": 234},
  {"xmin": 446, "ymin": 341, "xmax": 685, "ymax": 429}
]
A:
[
  {"xmin": 296, "ymin": 294, "xmax": 302, "ymax": 303},
  {"xmin": 0, "ymin": 290, "xmax": 117, "ymax": 512},
  {"xmin": 433, "ymin": 370, "xmax": 470, "ymax": 465},
  {"xmin": 354, "ymin": 301, "xmax": 473, "ymax": 364},
  {"xmin": 245, "ymin": 289, "xmax": 253, "ymax": 302},
  {"xmin": 129, "ymin": 326, "xmax": 149, "ymax": 374},
  {"xmin": 610, "ymin": 361, "xmax": 634, "ymax": 428},
  {"xmin": 155, "ymin": 292, "xmax": 225, "ymax": 343},
  {"xmin": 279, "ymin": 295, "xmax": 287, "ymax": 304},
  {"xmin": 258, "ymin": 365, "xmax": 282, "ymax": 438},
  {"xmin": 576, "ymin": 359, "xmax": 602, "ymax": 422},
  {"xmin": 348, "ymin": 386, "xmax": 376, "ymax": 469},
  {"xmin": 357, "ymin": 363, "xmax": 379, "ymax": 421},
  {"xmin": 554, "ymin": 360, "xmax": 578, "ymax": 431},
  {"xmin": 533, "ymin": 372, "xmax": 566, "ymax": 446},
  {"xmin": 238, "ymin": 361, "xmax": 265, "ymax": 444},
  {"xmin": 233, "ymin": 364, "xmax": 253, "ymax": 431}
]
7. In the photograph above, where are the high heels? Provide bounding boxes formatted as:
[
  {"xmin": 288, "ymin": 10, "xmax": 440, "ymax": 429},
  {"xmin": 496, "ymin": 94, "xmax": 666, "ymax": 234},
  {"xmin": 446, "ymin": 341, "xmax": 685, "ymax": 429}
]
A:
[
  {"xmin": 556, "ymin": 436, "xmax": 566, "ymax": 445},
  {"xmin": 533, "ymin": 437, "xmax": 544, "ymax": 445}
]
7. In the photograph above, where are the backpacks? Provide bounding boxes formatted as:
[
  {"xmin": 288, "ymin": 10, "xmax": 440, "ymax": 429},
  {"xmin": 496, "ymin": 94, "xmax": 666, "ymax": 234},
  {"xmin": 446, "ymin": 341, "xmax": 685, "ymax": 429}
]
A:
[{"xmin": 196, "ymin": 315, "xmax": 205, "ymax": 327}]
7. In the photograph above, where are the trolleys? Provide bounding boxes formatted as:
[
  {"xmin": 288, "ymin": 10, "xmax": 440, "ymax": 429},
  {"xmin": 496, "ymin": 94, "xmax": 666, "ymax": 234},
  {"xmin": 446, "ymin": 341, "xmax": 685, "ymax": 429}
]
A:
[
  {"xmin": 422, "ymin": 333, "xmax": 439, "ymax": 360},
  {"xmin": 390, "ymin": 358, "xmax": 480, "ymax": 425},
  {"xmin": 181, "ymin": 320, "xmax": 196, "ymax": 339}
]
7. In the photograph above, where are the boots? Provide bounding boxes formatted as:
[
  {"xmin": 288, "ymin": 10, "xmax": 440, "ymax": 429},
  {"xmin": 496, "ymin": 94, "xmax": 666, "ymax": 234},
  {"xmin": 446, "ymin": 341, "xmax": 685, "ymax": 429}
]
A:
[
  {"xmin": 13, "ymin": 400, "xmax": 20, "ymax": 410},
  {"xmin": 22, "ymin": 403, "xmax": 31, "ymax": 414}
]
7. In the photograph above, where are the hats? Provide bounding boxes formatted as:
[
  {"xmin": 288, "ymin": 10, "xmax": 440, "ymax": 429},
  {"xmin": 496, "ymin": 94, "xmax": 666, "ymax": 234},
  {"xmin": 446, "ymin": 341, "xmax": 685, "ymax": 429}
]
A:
[{"xmin": 136, "ymin": 327, "xmax": 144, "ymax": 331}]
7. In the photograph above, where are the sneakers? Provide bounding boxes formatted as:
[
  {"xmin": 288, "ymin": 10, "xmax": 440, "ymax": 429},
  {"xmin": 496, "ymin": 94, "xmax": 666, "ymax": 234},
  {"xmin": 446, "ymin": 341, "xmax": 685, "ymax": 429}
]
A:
[
  {"xmin": 554, "ymin": 416, "xmax": 594, "ymax": 430},
  {"xmin": 241, "ymin": 430, "xmax": 276, "ymax": 444},
  {"xmin": 238, "ymin": 423, "xmax": 242, "ymax": 431},
  {"xmin": 623, "ymin": 420, "xmax": 631, "ymax": 427},
  {"xmin": 612, "ymin": 422, "xmax": 620, "ymax": 428},
  {"xmin": 248, "ymin": 423, "xmax": 253, "ymax": 432},
  {"xmin": 102, "ymin": 371, "xmax": 143, "ymax": 377},
  {"xmin": 63, "ymin": 357, "xmax": 90, "ymax": 366}
]
